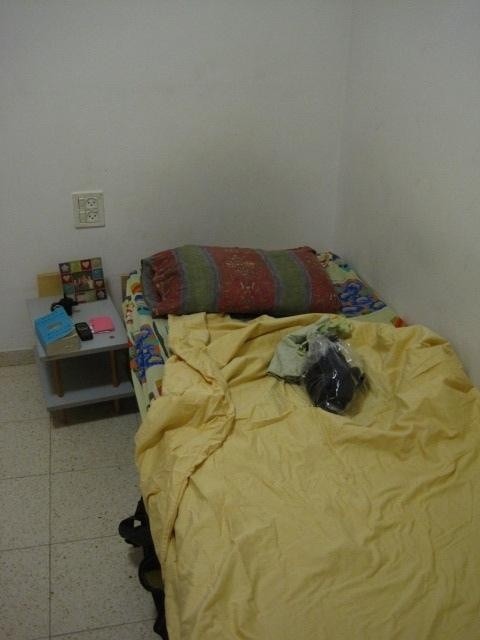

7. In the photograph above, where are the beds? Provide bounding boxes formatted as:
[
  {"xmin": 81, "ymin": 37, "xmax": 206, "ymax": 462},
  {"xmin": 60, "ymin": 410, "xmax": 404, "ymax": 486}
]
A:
[{"xmin": 119, "ymin": 245, "xmax": 480, "ymax": 640}]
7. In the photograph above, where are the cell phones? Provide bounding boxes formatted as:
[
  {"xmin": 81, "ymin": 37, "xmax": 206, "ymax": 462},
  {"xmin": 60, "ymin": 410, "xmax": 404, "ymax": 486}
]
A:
[{"xmin": 75, "ymin": 322, "xmax": 93, "ymax": 341}]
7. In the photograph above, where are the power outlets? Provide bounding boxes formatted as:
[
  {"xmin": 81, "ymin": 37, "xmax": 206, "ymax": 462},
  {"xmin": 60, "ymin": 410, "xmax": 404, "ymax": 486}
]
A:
[{"xmin": 71, "ymin": 191, "xmax": 105, "ymax": 228}]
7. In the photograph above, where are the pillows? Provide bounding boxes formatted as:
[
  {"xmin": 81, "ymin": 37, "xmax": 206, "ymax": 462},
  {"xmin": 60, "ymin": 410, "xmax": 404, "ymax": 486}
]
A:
[{"xmin": 141, "ymin": 245, "xmax": 341, "ymax": 317}]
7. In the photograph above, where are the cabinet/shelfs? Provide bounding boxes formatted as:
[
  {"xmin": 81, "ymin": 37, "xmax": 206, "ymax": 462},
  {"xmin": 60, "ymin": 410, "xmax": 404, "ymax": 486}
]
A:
[{"xmin": 25, "ymin": 291, "xmax": 135, "ymax": 425}]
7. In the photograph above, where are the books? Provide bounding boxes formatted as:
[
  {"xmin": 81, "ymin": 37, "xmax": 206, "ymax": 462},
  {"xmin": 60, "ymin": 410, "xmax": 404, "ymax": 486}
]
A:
[{"xmin": 34, "ymin": 306, "xmax": 82, "ymax": 356}]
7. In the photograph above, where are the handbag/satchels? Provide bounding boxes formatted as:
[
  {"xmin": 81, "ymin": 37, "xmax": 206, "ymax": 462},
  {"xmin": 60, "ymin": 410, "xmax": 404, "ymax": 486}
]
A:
[{"xmin": 305, "ymin": 346, "xmax": 368, "ymax": 416}]
